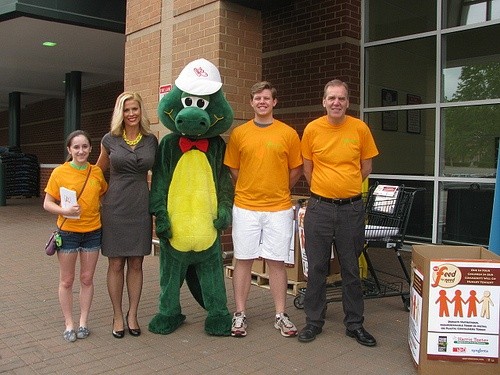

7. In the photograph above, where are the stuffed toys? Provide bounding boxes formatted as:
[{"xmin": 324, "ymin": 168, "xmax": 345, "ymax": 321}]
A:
[{"xmin": 149, "ymin": 57, "xmax": 234, "ymax": 336}]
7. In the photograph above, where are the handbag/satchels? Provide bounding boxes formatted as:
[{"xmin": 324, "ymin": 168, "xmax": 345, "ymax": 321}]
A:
[{"xmin": 44, "ymin": 230, "xmax": 62, "ymax": 255}]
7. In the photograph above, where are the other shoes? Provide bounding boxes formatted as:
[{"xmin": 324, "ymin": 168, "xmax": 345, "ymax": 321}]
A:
[
  {"xmin": 77, "ymin": 327, "xmax": 88, "ymax": 338},
  {"xmin": 63, "ymin": 329, "xmax": 77, "ymax": 342}
]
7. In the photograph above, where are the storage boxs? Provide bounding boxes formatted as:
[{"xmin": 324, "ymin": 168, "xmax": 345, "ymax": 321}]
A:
[
  {"xmin": 251, "ymin": 195, "xmax": 338, "ymax": 282},
  {"xmin": 407, "ymin": 245, "xmax": 500, "ymax": 375}
]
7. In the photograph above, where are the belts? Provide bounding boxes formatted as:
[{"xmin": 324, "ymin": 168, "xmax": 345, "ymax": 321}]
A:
[{"xmin": 311, "ymin": 191, "xmax": 362, "ymax": 205}]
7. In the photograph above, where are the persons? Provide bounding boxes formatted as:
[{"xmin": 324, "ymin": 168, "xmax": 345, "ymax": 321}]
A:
[
  {"xmin": 298, "ymin": 79, "xmax": 379, "ymax": 345},
  {"xmin": 43, "ymin": 130, "xmax": 108, "ymax": 342},
  {"xmin": 95, "ymin": 91, "xmax": 158, "ymax": 338},
  {"xmin": 224, "ymin": 80, "xmax": 304, "ymax": 338}
]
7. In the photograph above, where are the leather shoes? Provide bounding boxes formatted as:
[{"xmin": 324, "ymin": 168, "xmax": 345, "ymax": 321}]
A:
[
  {"xmin": 298, "ymin": 325, "xmax": 322, "ymax": 341},
  {"xmin": 346, "ymin": 326, "xmax": 376, "ymax": 346}
]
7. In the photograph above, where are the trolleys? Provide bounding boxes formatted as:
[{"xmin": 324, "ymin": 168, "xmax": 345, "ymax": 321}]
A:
[{"xmin": 292, "ymin": 181, "xmax": 428, "ymax": 309}]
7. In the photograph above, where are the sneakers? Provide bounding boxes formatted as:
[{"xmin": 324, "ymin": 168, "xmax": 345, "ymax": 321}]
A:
[
  {"xmin": 230, "ymin": 311, "xmax": 247, "ymax": 336},
  {"xmin": 274, "ymin": 313, "xmax": 298, "ymax": 336}
]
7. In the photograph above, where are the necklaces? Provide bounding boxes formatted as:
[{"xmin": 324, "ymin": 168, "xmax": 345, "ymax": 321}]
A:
[{"xmin": 122, "ymin": 127, "xmax": 142, "ymax": 146}]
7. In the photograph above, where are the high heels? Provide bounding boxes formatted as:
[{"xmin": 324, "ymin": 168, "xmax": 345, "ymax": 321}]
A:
[
  {"xmin": 125, "ymin": 311, "xmax": 141, "ymax": 336},
  {"xmin": 112, "ymin": 317, "xmax": 124, "ymax": 338}
]
